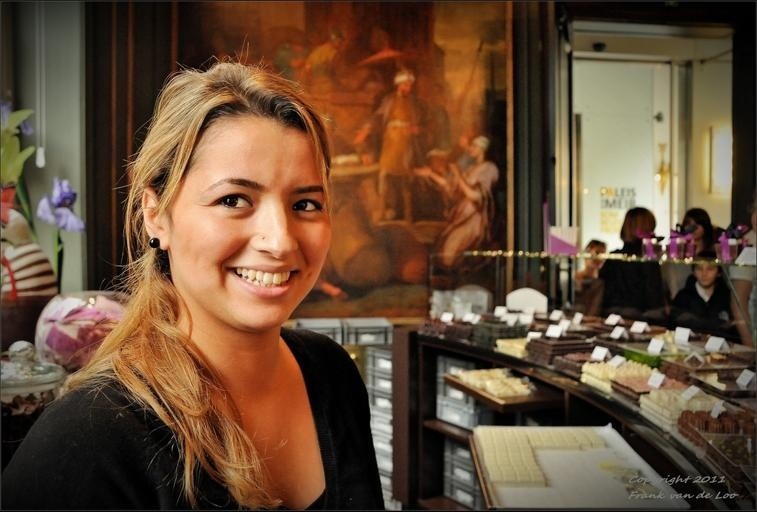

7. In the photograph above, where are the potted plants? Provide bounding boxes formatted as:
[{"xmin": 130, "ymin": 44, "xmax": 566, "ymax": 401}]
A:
[{"xmin": 1, "ymin": 107, "xmax": 37, "ymax": 226}]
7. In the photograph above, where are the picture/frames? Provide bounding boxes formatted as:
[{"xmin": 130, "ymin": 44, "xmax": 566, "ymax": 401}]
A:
[
  {"xmin": 705, "ymin": 125, "xmax": 732, "ymax": 197},
  {"xmin": 83, "ymin": 0, "xmax": 546, "ymax": 326}
]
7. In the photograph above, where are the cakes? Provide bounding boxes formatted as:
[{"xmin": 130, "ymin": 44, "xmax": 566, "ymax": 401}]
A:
[
  {"xmin": 494, "ymin": 337, "xmax": 537, "ymax": 357},
  {"xmin": 473, "ymin": 322, "xmax": 525, "ymax": 348},
  {"xmin": 445, "ymin": 321, "xmax": 493, "ymax": 343},
  {"xmin": 527, "ymin": 336, "xmax": 591, "ymax": 365},
  {"xmin": 611, "ymin": 376, "xmax": 685, "ymax": 401},
  {"xmin": 553, "ymin": 352, "xmax": 613, "ymax": 377},
  {"xmin": 419, "ymin": 319, "xmax": 453, "ymax": 337},
  {"xmin": 639, "ymin": 388, "xmax": 727, "ymax": 426},
  {"xmin": 682, "ymin": 409, "xmax": 756, "ymax": 434},
  {"xmin": 589, "ymin": 319, "xmax": 639, "ymax": 333},
  {"xmin": 454, "ymin": 369, "xmax": 535, "ymax": 398},
  {"xmin": 661, "ymin": 353, "xmax": 747, "ymax": 380},
  {"xmin": 475, "ymin": 425, "xmax": 606, "ymax": 484},
  {"xmin": 596, "ymin": 335, "xmax": 652, "ymax": 357},
  {"xmin": 565, "ymin": 327, "xmax": 610, "ymax": 344},
  {"xmin": 625, "ymin": 341, "xmax": 691, "ymax": 366},
  {"xmin": 581, "ymin": 360, "xmax": 655, "ymax": 391},
  {"xmin": 692, "ymin": 369, "xmax": 757, "ymax": 397},
  {"xmin": 629, "ymin": 325, "xmax": 666, "ymax": 340}
]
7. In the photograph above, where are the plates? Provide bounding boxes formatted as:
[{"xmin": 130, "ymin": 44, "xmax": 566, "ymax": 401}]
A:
[
  {"xmin": 678, "ymin": 414, "xmax": 757, "ymax": 467},
  {"xmin": 685, "ymin": 367, "xmax": 757, "ymax": 396},
  {"xmin": 594, "ymin": 333, "xmax": 665, "ymax": 352},
  {"xmin": 618, "ymin": 343, "xmax": 708, "ymax": 366},
  {"xmin": 656, "ymin": 354, "xmax": 756, "ymax": 382},
  {"xmin": 739, "ymin": 463, "xmax": 757, "ymax": 486}
]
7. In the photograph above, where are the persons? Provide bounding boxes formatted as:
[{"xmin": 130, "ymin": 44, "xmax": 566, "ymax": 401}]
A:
[
  {"xmin": 730, "ymin": 242, "xmax": 755, "ymax": 347},
  {"xmin": 360, "ymin": 71, "xmax": 424, "ymax": 224},
  {"xmin": 303, "ymin": 28, "xmax": 346, "ymax": 155},
  {"xmin": 575, "ymin": 241, "xmax": 606, "ymax": 313},
  {"xmin": 592, "ymin": 207, "xmax": 672, "ymax": 327},
  {"xmin": 0, "ymin": 62, "xmax": 384, "ymax": 511},
  {"xmin": 670, "ymin": 248, "xmax": 749, "ymax": 343},
  {"xmin": 681, "ymin": 208, "xmax": 719, "ymax": 259},
  {"xmin": 414, "ymin": 137, "xmax": 499, "ymax": 268}
]
